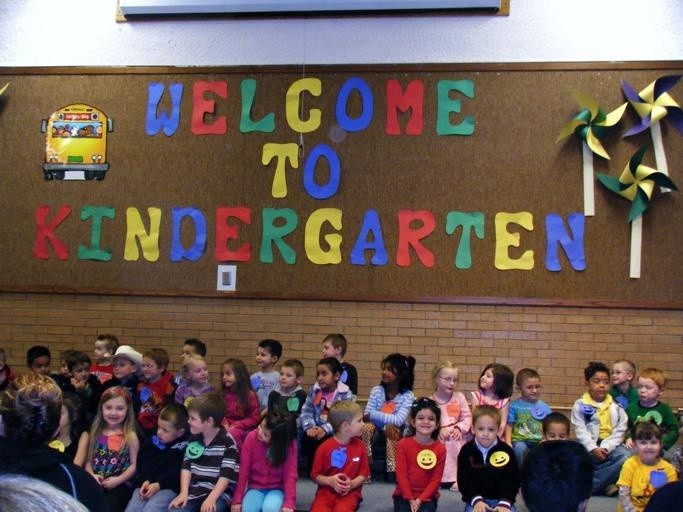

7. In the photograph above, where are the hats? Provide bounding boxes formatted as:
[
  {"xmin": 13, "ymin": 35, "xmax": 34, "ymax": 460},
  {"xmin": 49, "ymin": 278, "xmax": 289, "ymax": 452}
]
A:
[{"xmin": 105, "ymin": 345, "xmax": 143, "ymax": 366}]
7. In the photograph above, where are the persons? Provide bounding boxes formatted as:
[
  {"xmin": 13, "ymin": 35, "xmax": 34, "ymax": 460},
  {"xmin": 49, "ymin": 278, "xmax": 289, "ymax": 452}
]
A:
[
  {"xmin": 456, "ymin": 404, "xmax": 520, "ymax": 511},
  {"xmin": 571, "ymin": 358, "xmax": 682, "ymax": 512},
  {"xmin": 0, "ymin": 333, "xmax": 118, "ymax": 470},
  {"xmin": 392, "ymin": 397, "xmax": 446, "ymax": 512},
  {"xmin": 542, "ymin": 412, "xmax": 570, "ymax": 441},
  {"xmin": 84, "ymin": 332, "xmax": 371, "ymax": 511},
  {"xmin": 0, "ymin": 370, "xmax": 113, "ymax": 511},
  {"xmin": 365, "ymin": 353, "xmax": 416, "ymax": 484},
  {"xmin": 425, "ymin": 361, "xmax": 473, "ymax": 492},
  {"xmin": 470, "ymin": 363, "xmax": 552, "ymax": 470},
  {"xmin": 521, "ymin": 439, "xmax": 594, "ymax": 512}
]
